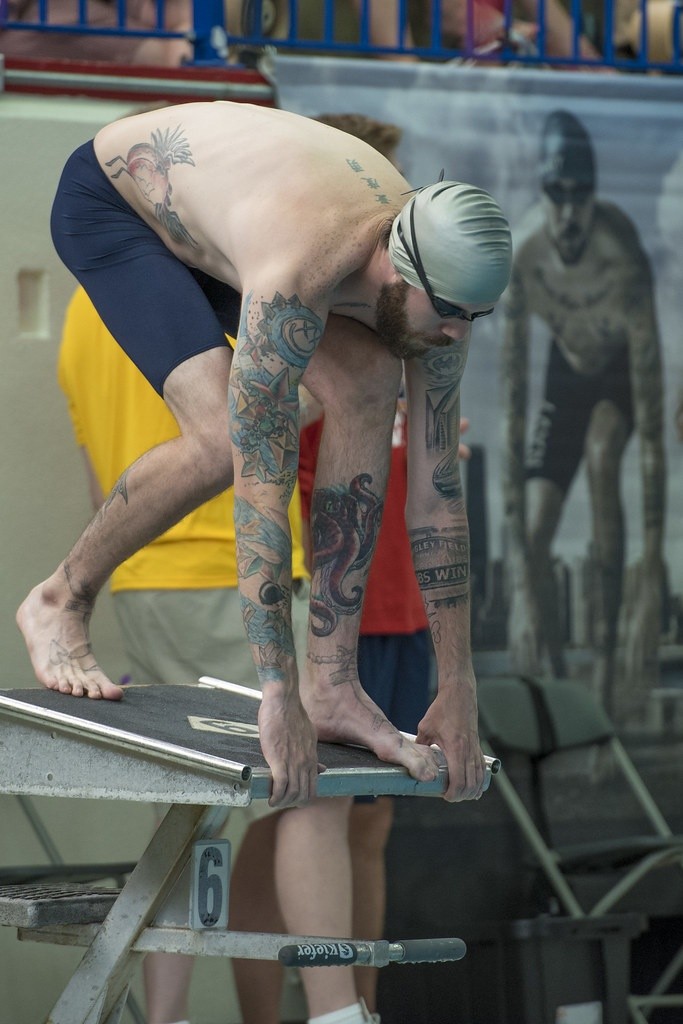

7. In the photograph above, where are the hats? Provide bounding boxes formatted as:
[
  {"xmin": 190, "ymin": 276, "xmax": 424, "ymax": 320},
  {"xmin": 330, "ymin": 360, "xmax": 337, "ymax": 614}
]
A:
[
  {"xmin": 388, "ymin": 180, "xmax": 512, "ymax": 305},
  {"xmin": 537, "ymin": 111, "xmax": 595, "ymax": 176}
]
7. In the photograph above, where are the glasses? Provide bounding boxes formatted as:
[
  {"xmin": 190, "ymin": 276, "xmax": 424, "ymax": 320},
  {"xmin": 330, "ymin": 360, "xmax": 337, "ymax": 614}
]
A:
[
  {"xmin": 415, "ymin": 269, "xmax": 494, "ymax": 322},
  {"xmin": 541, "ymin": 181, "xmax": 595, "ymax": 205}
]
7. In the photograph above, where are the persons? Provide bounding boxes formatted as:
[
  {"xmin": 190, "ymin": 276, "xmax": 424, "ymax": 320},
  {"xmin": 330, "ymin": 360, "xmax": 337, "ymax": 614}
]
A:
[
  {"xmin": 502, "ymin": 110, "xmax": 670, "ymax": 786},
  {"xmin": 0, "ymin": 1, "xmax": 683, "ymax": 61},
  {"xmin": 58, "ymin": 112, "xmax": 438, "ymax": 1024},
  {"xmin": 15, "ymin": 99, "xmax": 511, "ymax": 805}
]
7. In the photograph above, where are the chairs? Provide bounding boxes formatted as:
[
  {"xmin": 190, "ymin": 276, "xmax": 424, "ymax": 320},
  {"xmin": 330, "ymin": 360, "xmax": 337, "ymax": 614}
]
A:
[{"xmin": 442, "ymin": 674, "xmax": 683, "ymax": 1024}]
